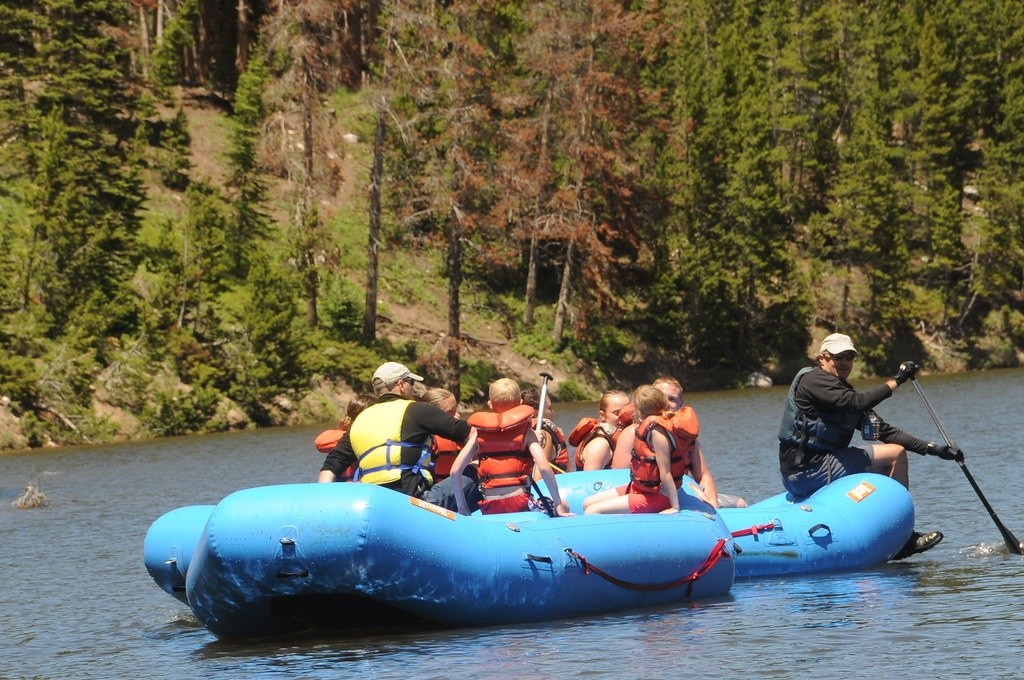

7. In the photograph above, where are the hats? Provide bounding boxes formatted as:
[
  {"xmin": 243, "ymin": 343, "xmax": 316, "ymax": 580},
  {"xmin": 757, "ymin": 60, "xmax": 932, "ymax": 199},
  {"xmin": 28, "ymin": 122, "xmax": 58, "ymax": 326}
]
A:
[
  {"xmin": 371, "ymin": 362, "xmax": 424, "ymax": 389},
  {"xmin": 820, "ymin": 333, "xmax": 858, "ymax": 354}
]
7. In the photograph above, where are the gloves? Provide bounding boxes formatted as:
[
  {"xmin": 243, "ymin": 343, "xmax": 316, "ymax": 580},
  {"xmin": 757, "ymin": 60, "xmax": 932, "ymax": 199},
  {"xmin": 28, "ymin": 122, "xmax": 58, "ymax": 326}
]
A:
[
  {"xmin": 893, "ymin": 361, "xmax": 919, "ymax": 386},
  {"xmin": 935, "ymin": 445, "xmax": 964, "ymax": 462}
]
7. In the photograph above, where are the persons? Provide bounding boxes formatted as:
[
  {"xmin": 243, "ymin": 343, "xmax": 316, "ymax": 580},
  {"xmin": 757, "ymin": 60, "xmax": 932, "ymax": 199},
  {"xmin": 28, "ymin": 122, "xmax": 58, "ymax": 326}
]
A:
[
  {"xmin": 777, "ymin": 332, "xmax": 965, "ymax": 560},
  {"xmin": 613, "ymin": 376, "xmax": 747, "ymax": 508},
  {"xmin": 413, "ymin": 381, "xmax": 460, "ymax": 483},
  {"xmin": 318, "ymin": 362, "xmax": 484, "ymax": 515},
  {"xmin": 583, "ymin": 386, "xmax": 699, "ymax": 514},
  {"xmin": 569, "ymin": 388, "xmax": 629, "ymax": 471},
  {"xmin": 315, "ymin": 394, "xmax": 378, "ymax": 482},
  {"xmin": 521, "ymin": 387, "xmax": 572, "ymax": 480},
  {"xmin": 451, "ymin": 378, "xmax": 577, "ymax": 517}
]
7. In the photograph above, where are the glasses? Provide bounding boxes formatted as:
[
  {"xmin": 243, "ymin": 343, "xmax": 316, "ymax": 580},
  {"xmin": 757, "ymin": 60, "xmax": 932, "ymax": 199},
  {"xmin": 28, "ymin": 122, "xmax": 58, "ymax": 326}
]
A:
[
  {"xmin": 822, "ymin": 352, "xmax": 855, "ymax": 360},
  {"xmin": 393, "ymin": 377, "xmax": 414, "ymax": 386}
]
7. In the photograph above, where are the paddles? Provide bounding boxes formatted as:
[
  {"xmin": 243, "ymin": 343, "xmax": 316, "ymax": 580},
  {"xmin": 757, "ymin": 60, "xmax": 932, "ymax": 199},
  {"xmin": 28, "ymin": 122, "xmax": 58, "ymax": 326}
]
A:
[
  {"xmin": 534, "ymin": 372, "xmax": 554, "ymax": 434},
  {"xmin": 911, "ymin": 375, "xmax": 1023, "ymax": 554}
]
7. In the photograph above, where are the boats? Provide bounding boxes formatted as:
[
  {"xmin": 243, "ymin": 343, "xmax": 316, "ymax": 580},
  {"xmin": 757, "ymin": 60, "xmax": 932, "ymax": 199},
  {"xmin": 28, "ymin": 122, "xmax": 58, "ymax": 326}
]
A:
[{"xmin": 144, "ymin": 467, "xmax": 915, "ymax": 636}]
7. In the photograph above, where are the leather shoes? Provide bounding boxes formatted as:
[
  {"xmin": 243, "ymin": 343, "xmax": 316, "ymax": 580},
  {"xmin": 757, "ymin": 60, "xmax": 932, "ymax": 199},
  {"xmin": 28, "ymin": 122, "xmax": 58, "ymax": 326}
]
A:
[{"xmin": 891, "ymin": 531, "xmax": 943, "ymax": 559}]
